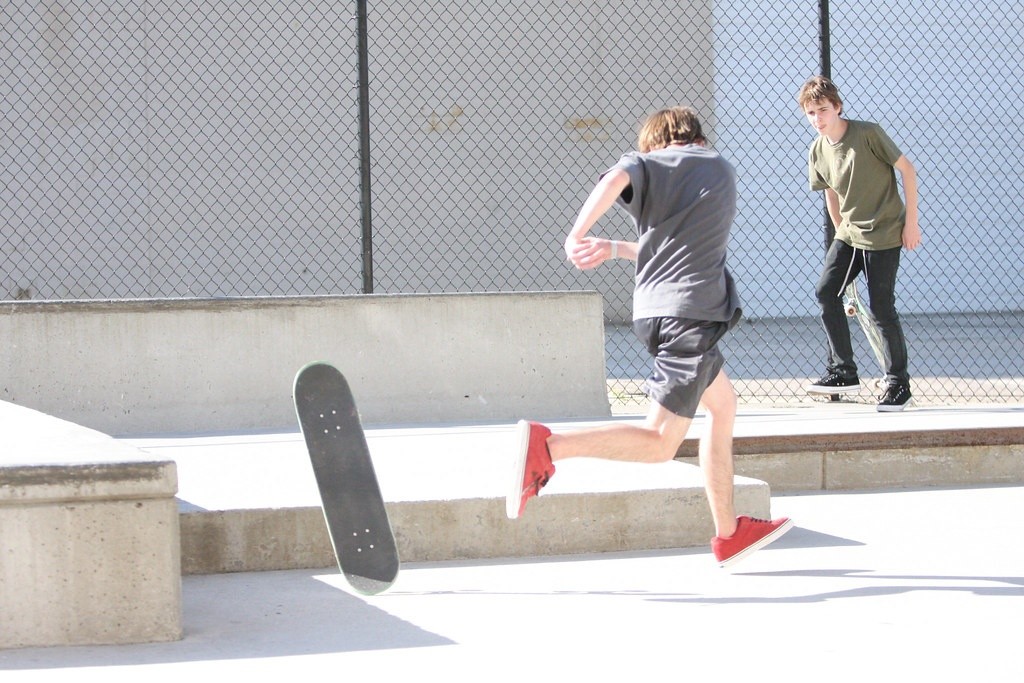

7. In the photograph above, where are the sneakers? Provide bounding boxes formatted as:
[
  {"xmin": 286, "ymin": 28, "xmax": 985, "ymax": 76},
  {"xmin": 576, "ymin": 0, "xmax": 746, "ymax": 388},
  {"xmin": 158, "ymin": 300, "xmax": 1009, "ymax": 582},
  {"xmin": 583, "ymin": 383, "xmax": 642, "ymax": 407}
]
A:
[
  {"xmin": 710, "ymin": 515, "xmax": 793, "ymax": 567},
  {"xmin": 875, "ymin": 383, "xmax": 912, "ymax": 412},
  {"xmin": 506, "ymin": 419, "xmax": 557, "ymax": 520},
  {"xmin": 807, "ymin": 366, "xmax": 860, "ymax": 394}
]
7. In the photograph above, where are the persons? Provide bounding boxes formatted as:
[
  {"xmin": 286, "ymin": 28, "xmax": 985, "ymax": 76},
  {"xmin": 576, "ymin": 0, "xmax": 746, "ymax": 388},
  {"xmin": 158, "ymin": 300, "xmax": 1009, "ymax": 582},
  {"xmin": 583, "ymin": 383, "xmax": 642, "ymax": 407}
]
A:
[
  {"xmin": 799, "ymin": 74, "xmax": 926, "ymax": 412},
  {"xmin": 507, "ymin": 107, "xmax": 799, "ymax": 570}
]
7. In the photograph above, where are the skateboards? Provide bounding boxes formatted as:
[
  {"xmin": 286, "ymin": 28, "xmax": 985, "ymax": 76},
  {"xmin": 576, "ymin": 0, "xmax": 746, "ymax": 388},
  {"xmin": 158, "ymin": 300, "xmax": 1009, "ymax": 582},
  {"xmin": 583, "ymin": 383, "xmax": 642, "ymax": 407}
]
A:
[
  {"xmin": 294, "ymin": 359, "xmax": 402, "ymax": 596},
  {"xmin": 844, "ymin": 279, "xmax": 886, "ymax": 390}
]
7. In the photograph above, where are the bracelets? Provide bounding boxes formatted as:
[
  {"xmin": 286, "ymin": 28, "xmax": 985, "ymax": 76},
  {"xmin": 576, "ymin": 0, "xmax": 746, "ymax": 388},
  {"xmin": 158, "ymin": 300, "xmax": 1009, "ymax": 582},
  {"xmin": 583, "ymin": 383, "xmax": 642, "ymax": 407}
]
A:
[{"xmin": 608, "ymin": 240, "xmax": 619, "ymax": 262}]
